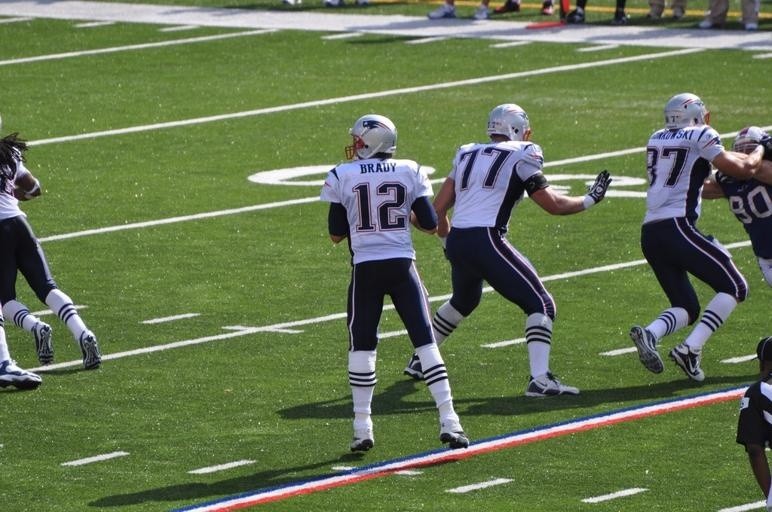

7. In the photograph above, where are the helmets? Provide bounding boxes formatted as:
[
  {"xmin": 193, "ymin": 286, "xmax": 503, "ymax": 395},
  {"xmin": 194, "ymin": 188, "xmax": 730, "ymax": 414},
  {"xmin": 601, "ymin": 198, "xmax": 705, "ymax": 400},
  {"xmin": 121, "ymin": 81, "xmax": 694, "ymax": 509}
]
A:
[
  {"xmin": 485, "ymin": 101, "xmax": 534, "ymax": 144},
  {"xmin": 663, "ymin": 92, "xmax": 714, "ymax": 132},
  {"xmin": 729, "ymin": 123, "xmax": 768, "ymax": 162},
  {"xmin": 343, "ymin": 110, "xmax": 399, "ymax": 161}
]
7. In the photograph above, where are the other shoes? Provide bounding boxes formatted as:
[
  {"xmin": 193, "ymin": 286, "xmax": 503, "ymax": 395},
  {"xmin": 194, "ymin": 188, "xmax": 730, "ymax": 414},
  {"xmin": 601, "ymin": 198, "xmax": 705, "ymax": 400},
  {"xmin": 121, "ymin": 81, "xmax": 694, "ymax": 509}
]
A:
[
  {"xmin": 647, "ymin": 12, "xmax": 660, "ymax": 20},
  {"xmin": 672, "ymin": 7, "xmax": 683, "ymax": 16},
  {"xmin": 427, "ymin": 5, "xmax": 455, "ymax": 20},
  {"xmin": 745, "ymin": 22, "xmax": 757, "ymax": 30},
  {"xmin": 612, "ymin": 11, "xmax": 628, "ymax": 26},
  {"xmin": 542, "ymin": 0, "xmax": 556, "ymax": 15},
  {"xmin": 568, "ymin": 9, "xmax": 585, "ymax": 24},
  {"xmin": 560, "ymin": 11, "xmax": 569, "ymax": 22},
  {"xmin": 471, "ymin": 5, "xmax": 490, "ymax": 19},
  {"xmin": 699, "ymin": 19, "xmax": 714, "ymax": 28},
  {"xmin": 494, "ymin": 0, "xmax": 521, "ymax": 13}
]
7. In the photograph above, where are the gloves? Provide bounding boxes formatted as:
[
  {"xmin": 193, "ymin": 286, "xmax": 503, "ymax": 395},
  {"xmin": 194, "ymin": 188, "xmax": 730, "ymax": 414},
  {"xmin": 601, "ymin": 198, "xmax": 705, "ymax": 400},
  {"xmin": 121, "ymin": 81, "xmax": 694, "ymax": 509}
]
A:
[{"xmin": 581, "ymin": 169, "xmax": 613, "ymax": 211}]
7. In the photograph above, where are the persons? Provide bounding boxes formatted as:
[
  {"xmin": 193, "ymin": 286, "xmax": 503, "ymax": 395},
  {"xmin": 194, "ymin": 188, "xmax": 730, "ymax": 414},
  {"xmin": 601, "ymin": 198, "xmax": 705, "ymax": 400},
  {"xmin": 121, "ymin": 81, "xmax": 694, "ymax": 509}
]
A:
[
  {"xmin": 404, "ymin": 102, "xmax": 613, "ymax": 397},
  {"xmin": 629, "ymin": 90, "xmax": 771, "ymax": 381},
  {"xmin": 701, "ymin": 126, "xmax": 771, "ymax": 284},
  {"xmin": 0, "ymin": 299, "xmax": 42, "ymax": 388},
  {"xmin": 322, "ymin": 113, "xmax": 470, "ymax": 453},
  {"xmin": 0, "ymin": 115, "xmax": 101, "ymax": 370},
  {"xmin": 737, "ymin": 336, "xmax": 771, "ymax": 511}
]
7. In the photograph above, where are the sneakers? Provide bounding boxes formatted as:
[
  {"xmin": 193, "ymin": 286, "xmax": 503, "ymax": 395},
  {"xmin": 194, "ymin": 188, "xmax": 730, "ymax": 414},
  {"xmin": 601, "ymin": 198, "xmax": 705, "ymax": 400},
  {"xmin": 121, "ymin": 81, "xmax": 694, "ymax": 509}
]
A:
[
  {"xmin": 521, "ymin": 369, "xmax": 582, "ymax": 401},
  {"xmin": 0, "ymin": 360, "xmax": 45, "ymax": 390},
  {"xmin": 668, "ymin": 340, "xmax": 708, "ymax": 383},
  {"xmin": 34, "ymin": 322, "xmax": 58, "ymax": 367},
  {"xmin": 627, "ymin": 324, "xmax": 667, "ymax": 377},
  {"xmin": 347, "ymin": 429, "xmax": 376, "ymax": 453},
  {"xmin": 436, "ymin": 428, "xmax": 471, "ymax": 452},
  {"xmin": 76, "ymin": 327, "xmax": 103, "ymax": 371},
  {"xmin": 401, "ymin": 354, "xmax": 425, "ymax": 380}
]
